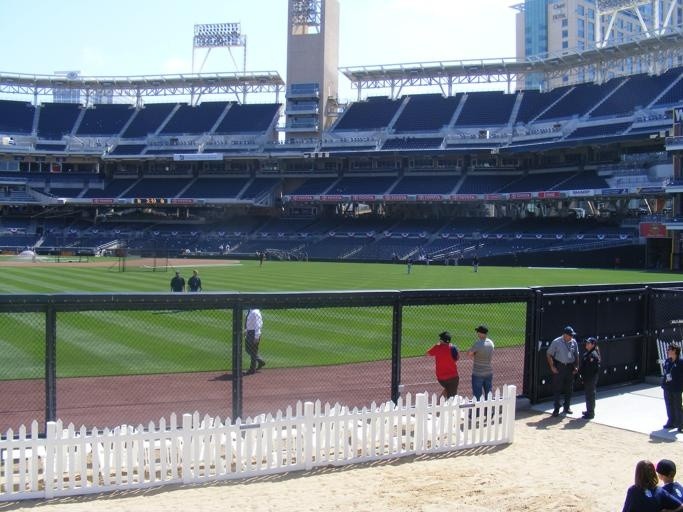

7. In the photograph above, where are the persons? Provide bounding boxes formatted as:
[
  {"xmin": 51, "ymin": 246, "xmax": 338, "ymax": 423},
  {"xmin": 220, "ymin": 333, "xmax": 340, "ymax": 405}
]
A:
[
  {"xmin": 621, "ymin": 459, "xmax": 683, "ymax": 512},
  {"xmin": 465, "ymin": 325, "xmax": 494, "ymax": 421},
  {"xmin": 655, "ymin": 459, "xmax": 683, "ymax": 502},
  {"xmin": 169, "ymin": 271, "xmax": 186, "ymax": 294},
  {"xmin": 187, "ymin": 270, "xmax": 202, "ymax": 292},
  {"xmin": 180, "ymin": 241, "xmax": 308, "ymax": 265},
  {"xmin": 580, "ymin": 337, "xmax": 601, "ymax": 419},
  {"xmin": 424, "ymin": 332, "xmax": 461, "ymax": 405},
  {"xmin": 545, "ymin": 326, "xmax": 579, "ymax": 417},
  {"xmin": 660, "ymin": 343, "xmax": 683, "ymax": 433},
  {"xmin": 242, "ymin": 309, "xmax": 264, "ymax": 377},
  {"xmin": 390, "ymin": 242, "xmax": 481, "ymax": 275}
]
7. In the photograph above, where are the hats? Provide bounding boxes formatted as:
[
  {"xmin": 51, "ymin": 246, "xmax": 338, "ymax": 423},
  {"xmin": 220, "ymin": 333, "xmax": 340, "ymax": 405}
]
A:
[
  {"xmin": 585, "ymin": 337, "xmax": 597, "ymax": 343},
  {"xmin": 475, "ymin": 325, "xmax": 488, "ymax": 333},
  {"xmin": 439, "ymin": 332, "xmax": 451, "ymax": 339},
  {"xmin": 654, "ymin": 459, "xmax": 676, "ymax": 477},
  {"xmin": 564, "ymin": 326, "xmax": 576, "ymax": 335}
]
